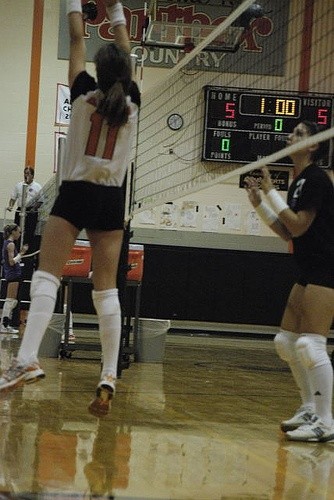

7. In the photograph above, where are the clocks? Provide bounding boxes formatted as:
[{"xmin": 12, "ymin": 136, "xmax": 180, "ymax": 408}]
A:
[{"xmin": 168, "ymin": 113, "xmax": 184, "ymax": 130}]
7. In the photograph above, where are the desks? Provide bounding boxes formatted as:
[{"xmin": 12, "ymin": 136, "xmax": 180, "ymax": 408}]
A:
[{"xmin": 60, "ymin": 276, "xmax": 142, "ymax": 361}]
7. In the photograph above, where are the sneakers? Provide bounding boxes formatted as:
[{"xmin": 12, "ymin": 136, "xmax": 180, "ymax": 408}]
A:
[
  {"xmin": 279, "ymin": 410, "xmax": 318, "ymax": 433},
  {"xmin": 0, "ymin": 323, "xmax": 19, "ymax": 334},
  {"xmin": 87, "ymin": 375, "xmax": 117, "ymax": 417},
  {"xmin": 0, "ymin": 358, "xmax": 45, "ymax": 393},
  {"xmin": 285, "ymin": 415, "xmax": 334, "ymax": 443}
]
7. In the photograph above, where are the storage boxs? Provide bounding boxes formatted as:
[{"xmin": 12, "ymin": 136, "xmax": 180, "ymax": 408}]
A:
[
  {"xmin": 126, "ymin": 244, "xmax": 145, "ymax": 280},
  {"xmin": 62, "ymin": 239, "xmax": 93, "ymax": 277}
]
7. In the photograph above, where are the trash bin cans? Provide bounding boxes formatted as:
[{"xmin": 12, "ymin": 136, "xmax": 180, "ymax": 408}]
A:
[{"xmin": 133, "ymin": 318, "xmax": 171, "ymax": 362}]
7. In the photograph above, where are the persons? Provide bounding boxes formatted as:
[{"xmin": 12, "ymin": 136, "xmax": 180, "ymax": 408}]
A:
[
  {"xmin": 244, "ymin": 117, "xmax": 334, "ymax": 443},
  {"xmin": 0, "ymin": 223, "xmax": 28, "ymax": 334},
  {"xmin": 0, "ymin": 0, "xmax": 140, "ymax": 416},
  {"xmin": 7, "ymin": 167, "xmax": 44, "ymax": 261}
]
